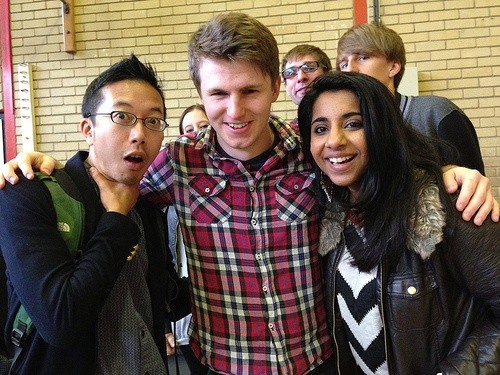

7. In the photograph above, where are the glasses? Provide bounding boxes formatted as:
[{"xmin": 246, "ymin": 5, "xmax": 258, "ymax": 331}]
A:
[
  {"xmin": 86, "ymin": 111, "xmax": 169, "ymax": 132},
  {"xmin": 282, "ymin": 61, "xmax": 328, "ymax": 78}
]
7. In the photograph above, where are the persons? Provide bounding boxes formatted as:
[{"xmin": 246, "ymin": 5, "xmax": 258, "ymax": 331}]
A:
[
  {"xmin": 281, "ymin": 45, "xmax": 332, "ymax": 106},
  {"xmin": 298, "ymin": 71, "xmax": 500, "ymax": 375},
  {"xmin": 164, "ymin": 104, "xmax": 211, "ymax": 374},
  {"xmin": 0, "ymin": 11, "xmax": 500, "ymax": 375},
  {"xmin": 1, "ymin": 53, "xmax": 196, "ymax": 375},
  {"xmin": 335, "ymin": 24, "xmax": 485, "ymax": 177}
]
